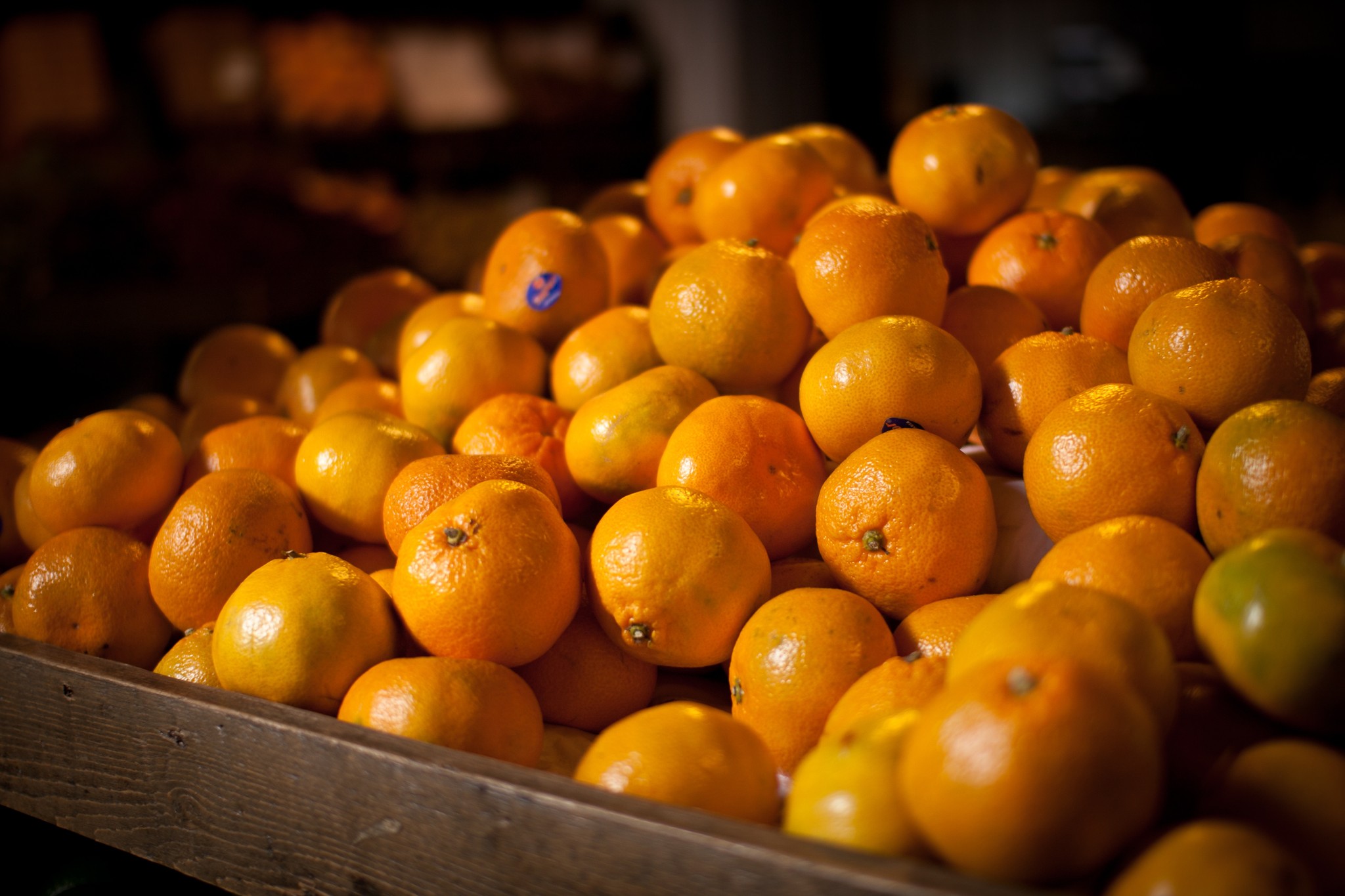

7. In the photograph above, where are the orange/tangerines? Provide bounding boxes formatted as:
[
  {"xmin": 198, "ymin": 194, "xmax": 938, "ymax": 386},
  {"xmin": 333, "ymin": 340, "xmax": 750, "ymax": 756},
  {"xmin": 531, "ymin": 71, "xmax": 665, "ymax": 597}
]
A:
[{"xmin": 0, "ymin": 105, "xmax": 1345, "ymax": 896}]
